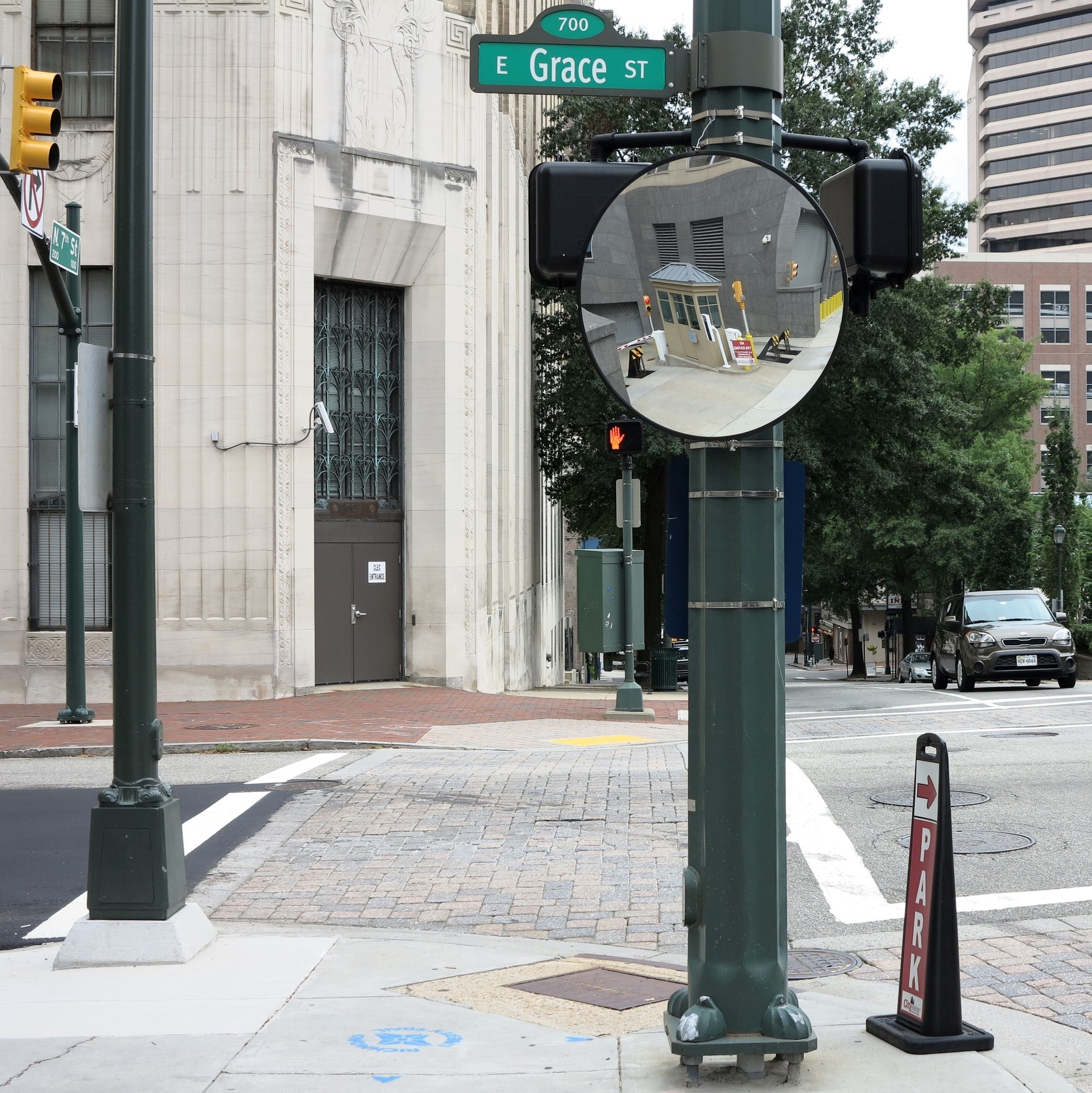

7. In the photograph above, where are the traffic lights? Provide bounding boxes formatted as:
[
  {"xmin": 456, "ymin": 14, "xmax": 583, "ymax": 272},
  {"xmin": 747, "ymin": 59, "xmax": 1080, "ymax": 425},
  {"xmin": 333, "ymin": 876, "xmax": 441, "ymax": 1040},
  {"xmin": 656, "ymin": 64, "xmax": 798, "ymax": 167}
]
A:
[
  {"xmin": 811, "ymin": 627, "xmax": 818, "ymax": 632},
  {"xmin": 787, "ymin": 261, "xmax": 798, "ymax": 282},
  {"xmin": 644, "ymin": 295, "xmax": 653, "ymax": 313},
  {"xmin": 732, "ymin": 281, "xmax": 744, "ymax": 303},
  {"xmin": 832, "ymin": 255, "xmax": 838, "ymax": 267},
  {"xmin": 606, "ymin": 420, "xmax": 643, "ymax": 454},
  {"xmin": 885, "ymin": 630, "xmax": 894, "ymax": 637},
  {"xmin": 10, "ymin": 64, "xmax": 63, "ymax": 172},
  {"xmin": 818, "ymin": 147, "xmax": 922, "ymax": 286}
]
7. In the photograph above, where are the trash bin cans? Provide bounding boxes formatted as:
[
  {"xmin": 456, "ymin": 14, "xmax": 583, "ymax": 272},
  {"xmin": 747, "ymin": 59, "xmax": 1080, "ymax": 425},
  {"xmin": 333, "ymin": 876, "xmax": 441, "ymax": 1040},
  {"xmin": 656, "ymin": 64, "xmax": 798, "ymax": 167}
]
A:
[{"xmin": 647, "ymin": 646, "xmax": 680, "ymax": 690}]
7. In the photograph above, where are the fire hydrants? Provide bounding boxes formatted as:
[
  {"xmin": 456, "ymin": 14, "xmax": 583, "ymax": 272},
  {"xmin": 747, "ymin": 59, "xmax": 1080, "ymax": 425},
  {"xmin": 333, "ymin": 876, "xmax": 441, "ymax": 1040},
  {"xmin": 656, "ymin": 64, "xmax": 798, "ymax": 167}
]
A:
[{"xmin": 808, "ymin": 657, "xmax": 813, "ymax": 667}]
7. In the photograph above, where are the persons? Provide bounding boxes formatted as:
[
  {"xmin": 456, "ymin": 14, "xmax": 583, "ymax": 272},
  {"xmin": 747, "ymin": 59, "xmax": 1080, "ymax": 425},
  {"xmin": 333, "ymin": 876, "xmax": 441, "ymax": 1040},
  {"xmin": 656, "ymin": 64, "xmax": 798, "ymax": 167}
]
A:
[
  {"xmin": 828, "ymin": 646, "xmax": 835, "ymax": 665},
  {"xmin": 785, "ymin": 643, "xmax": 788, "ymax": 654}
]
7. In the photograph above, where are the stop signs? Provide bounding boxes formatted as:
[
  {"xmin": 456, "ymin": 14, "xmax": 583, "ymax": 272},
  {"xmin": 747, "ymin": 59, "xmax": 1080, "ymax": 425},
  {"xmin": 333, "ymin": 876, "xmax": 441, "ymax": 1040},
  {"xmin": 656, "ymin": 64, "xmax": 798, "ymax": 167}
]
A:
[{"xmin": 812, "ymin": 634, "xmax": 819, "ymax": 642}]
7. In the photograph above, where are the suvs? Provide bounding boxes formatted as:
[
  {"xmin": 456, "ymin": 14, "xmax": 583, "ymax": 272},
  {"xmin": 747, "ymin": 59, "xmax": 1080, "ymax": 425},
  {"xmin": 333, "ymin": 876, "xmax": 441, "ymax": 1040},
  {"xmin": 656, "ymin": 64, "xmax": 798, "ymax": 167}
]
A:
[{"xmin": 932, "ymin": 589, "xmax": 1076, "ymax": 692}]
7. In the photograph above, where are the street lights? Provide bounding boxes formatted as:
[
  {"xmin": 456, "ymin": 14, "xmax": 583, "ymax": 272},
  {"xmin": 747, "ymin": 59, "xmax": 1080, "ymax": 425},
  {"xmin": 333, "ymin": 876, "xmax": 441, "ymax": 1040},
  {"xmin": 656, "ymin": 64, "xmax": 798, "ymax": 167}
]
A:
[{"xmin": 1054, "ymin": 526, "xmax": 1066, "ymax": 624}]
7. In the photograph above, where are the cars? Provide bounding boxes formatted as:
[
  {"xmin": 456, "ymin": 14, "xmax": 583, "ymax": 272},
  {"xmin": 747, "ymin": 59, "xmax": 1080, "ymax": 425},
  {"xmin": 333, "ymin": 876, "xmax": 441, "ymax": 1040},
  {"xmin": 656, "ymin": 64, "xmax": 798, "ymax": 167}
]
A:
[{"xmin": 897, "ymin": 652, "xmax": 953, "ymax": 683}]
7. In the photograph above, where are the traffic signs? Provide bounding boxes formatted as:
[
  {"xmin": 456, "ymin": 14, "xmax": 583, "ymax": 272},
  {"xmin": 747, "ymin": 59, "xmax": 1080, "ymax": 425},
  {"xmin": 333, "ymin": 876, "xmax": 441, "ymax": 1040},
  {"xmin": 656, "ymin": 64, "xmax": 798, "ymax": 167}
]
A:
[{"xmin": 898, "ymin": 759, "xmax": 940, "ymax": 1024}]
